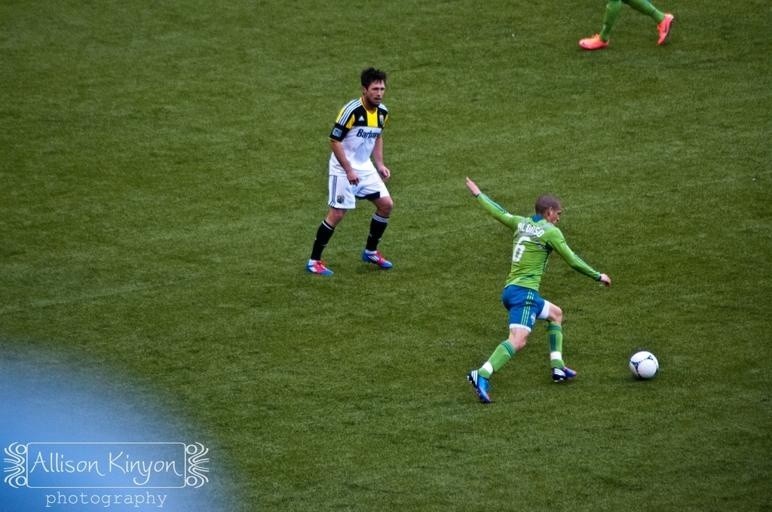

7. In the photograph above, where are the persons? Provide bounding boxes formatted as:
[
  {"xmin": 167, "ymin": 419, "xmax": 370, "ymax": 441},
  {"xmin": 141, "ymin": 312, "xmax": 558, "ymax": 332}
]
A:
[
  {"xmin": 577, "ymin": 0, "xmax": 674, "ymax": 49},
  {"xmin": 306, "ymin": 68, "xmax": 395, "ymax": 275},
  {"xmin": 464, "ymin": 176, "xmax": 611, "ymax": 404}
]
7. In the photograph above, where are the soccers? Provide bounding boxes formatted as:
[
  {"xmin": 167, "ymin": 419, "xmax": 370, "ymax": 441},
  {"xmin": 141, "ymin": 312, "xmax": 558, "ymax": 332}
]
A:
[{"xmin": 629, "ymin": 350, "xmax": 659, "ymax": 380}]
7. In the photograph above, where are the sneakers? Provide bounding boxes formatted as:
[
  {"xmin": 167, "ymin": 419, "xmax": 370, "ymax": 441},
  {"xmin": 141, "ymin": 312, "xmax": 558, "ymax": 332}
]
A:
[
  {"xmin": 552, "ymin": 368, "xmax": 577, "ymax": 382},
  {"xmin": 363, "ymin": 249, "xmax": 393, "ymax": 268},
  {"xmin": 656, "ymin": 13, "xmax": 674, "ymax": 46},
  {"xmin": 305, "ymin": 258, "xmax": 334, "ymax": 276},
  {"xmin": 579, "ymin": 33, "xmax": 608, "ymax": 49},
  {"xmin": 467, "ymin": 369, "xmax": 490, "ymax": 404}
]
